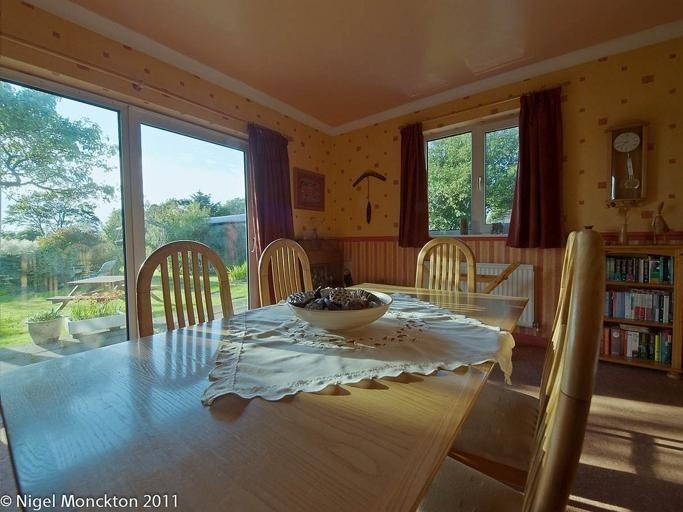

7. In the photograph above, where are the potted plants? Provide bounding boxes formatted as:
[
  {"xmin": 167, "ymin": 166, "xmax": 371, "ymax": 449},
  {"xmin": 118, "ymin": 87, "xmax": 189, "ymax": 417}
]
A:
[
  {"xmin": 26, "ymin": 309, "xmax": 61, "ymax": 344},
  {"xmin": 69, "ymin": 302, "xmax": 125, "ymax": 339}
]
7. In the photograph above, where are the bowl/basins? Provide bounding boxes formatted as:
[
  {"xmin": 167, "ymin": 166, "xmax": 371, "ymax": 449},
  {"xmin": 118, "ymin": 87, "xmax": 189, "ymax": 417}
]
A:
[{"xmin": 287, "ymin": 289, "xmax": 394, "ymax": 335}]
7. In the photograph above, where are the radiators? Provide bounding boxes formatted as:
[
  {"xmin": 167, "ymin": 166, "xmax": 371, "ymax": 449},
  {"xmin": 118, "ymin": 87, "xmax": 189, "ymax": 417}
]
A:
[{"xmin": 423, "ymin": 261, "xmax": 540, "ymax": 334}]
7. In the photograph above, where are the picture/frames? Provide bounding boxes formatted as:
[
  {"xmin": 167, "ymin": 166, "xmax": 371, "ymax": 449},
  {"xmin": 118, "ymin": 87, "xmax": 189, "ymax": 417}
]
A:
[{"xmin": 294, "ymin": 166, "xmax": 325, "ymax": 211}]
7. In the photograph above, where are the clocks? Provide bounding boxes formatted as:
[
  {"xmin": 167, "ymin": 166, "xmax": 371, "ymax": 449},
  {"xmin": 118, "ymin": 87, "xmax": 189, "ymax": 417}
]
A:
[{"xmin": 604, "ymin": 118, "xmax": 649, "ymax": 208}]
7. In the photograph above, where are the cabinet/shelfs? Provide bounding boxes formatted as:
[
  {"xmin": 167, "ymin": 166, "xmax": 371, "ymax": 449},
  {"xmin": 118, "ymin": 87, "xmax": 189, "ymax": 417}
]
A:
[{"xmin": 598, "ymin": 245, "xmax": 683, "ymax": 380}]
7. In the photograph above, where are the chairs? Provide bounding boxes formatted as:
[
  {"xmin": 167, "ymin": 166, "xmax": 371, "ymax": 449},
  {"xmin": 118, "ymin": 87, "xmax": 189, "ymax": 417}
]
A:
[
  {"xmin": 417, "ymin": 230, "xmax": 608, "ymax": 512},
  {"xmin": 448, "ymin": 230, "xmax": 577, "ymax": 490},
  {"xmin": 415, "ymin": 237, "xmax": 475, "ymax": 293},
  {"xmin": 136, "ymin": 240, "xmax": 234, "ymax": 338},
  {"xmin": 257, "ymin": 238, "xmax": 313, "ymax": 307}
]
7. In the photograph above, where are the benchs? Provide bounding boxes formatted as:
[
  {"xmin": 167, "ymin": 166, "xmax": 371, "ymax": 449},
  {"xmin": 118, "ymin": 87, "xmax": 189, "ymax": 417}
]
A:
[
  {"xmin": 45, "ymin": 295, "xmax": 111, "ymax": 316},
  {"xmin": 117, "ymin": 284, "xmax": 162, "ymax": 304}
]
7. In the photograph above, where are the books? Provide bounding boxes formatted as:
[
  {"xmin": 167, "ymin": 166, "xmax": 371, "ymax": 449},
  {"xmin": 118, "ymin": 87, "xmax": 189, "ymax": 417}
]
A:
[{"xmin": 600, "ymin": 254, "xmax": 672, "ymax": 365}]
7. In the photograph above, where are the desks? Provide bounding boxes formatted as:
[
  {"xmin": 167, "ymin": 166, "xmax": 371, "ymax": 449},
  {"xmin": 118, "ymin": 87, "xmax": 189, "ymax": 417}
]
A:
[{"xmin": 65, "ymin": 275, "xmax": 126, "ymax": 297}]
